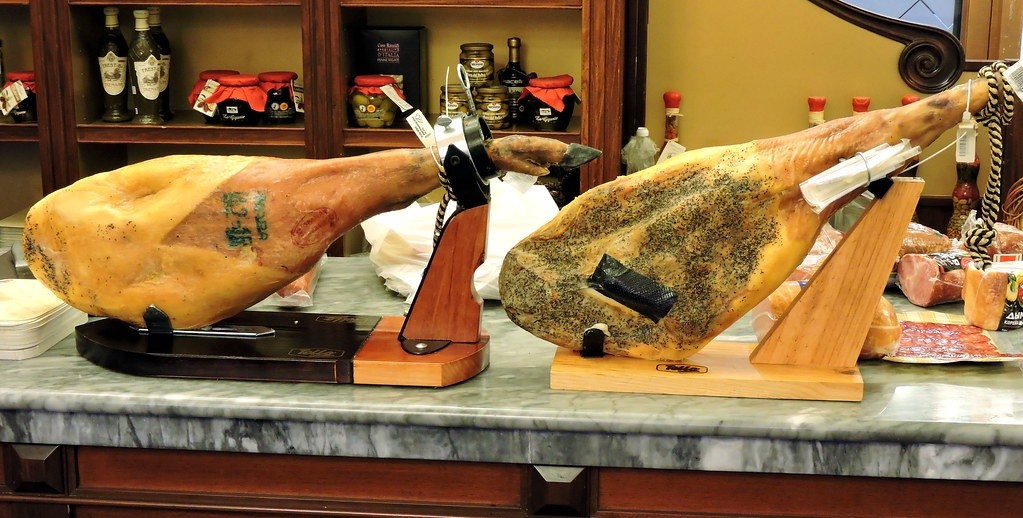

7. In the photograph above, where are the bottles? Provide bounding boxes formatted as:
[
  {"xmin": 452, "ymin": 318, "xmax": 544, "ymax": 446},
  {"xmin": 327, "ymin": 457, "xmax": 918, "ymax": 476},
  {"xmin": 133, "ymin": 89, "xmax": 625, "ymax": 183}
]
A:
[
  {"xmin": 147, "ymin": 6, "xmax": 170, "ymax": 119},
  {"xmin": 625, "ymin": 126, "xmax": 657, "ymax": 174},
  {"xmin": 96, "ymin": 6, "xmax": 129, "ymax": 122},
  {"xmin": 128, "ymin": 10, "xmax": 161, "ymax": 124},
  {"xmin": 499, "ymin": 38, "xmax": 527, "ymax": 120}
]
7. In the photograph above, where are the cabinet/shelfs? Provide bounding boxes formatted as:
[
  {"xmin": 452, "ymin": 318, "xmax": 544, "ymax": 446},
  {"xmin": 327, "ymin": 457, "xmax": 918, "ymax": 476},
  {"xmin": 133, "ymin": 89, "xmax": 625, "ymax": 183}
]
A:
[{"xmin": 0, "ymin": 1, "xmax": 648, "ymax": 198}]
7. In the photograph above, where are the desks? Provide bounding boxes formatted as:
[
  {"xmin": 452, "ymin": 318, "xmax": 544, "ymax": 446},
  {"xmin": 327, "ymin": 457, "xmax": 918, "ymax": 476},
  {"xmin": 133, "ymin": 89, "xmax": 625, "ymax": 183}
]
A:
[{"xmin": 0, "ymin": 254, "xmax": 1022, "ymax": 517}]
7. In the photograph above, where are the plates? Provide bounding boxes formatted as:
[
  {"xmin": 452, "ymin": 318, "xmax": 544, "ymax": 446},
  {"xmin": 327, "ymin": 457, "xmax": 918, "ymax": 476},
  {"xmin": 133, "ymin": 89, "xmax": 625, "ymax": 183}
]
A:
[{"xmin": 0, "ymin": 206, "xmax": 90, "ymax": 360}]
